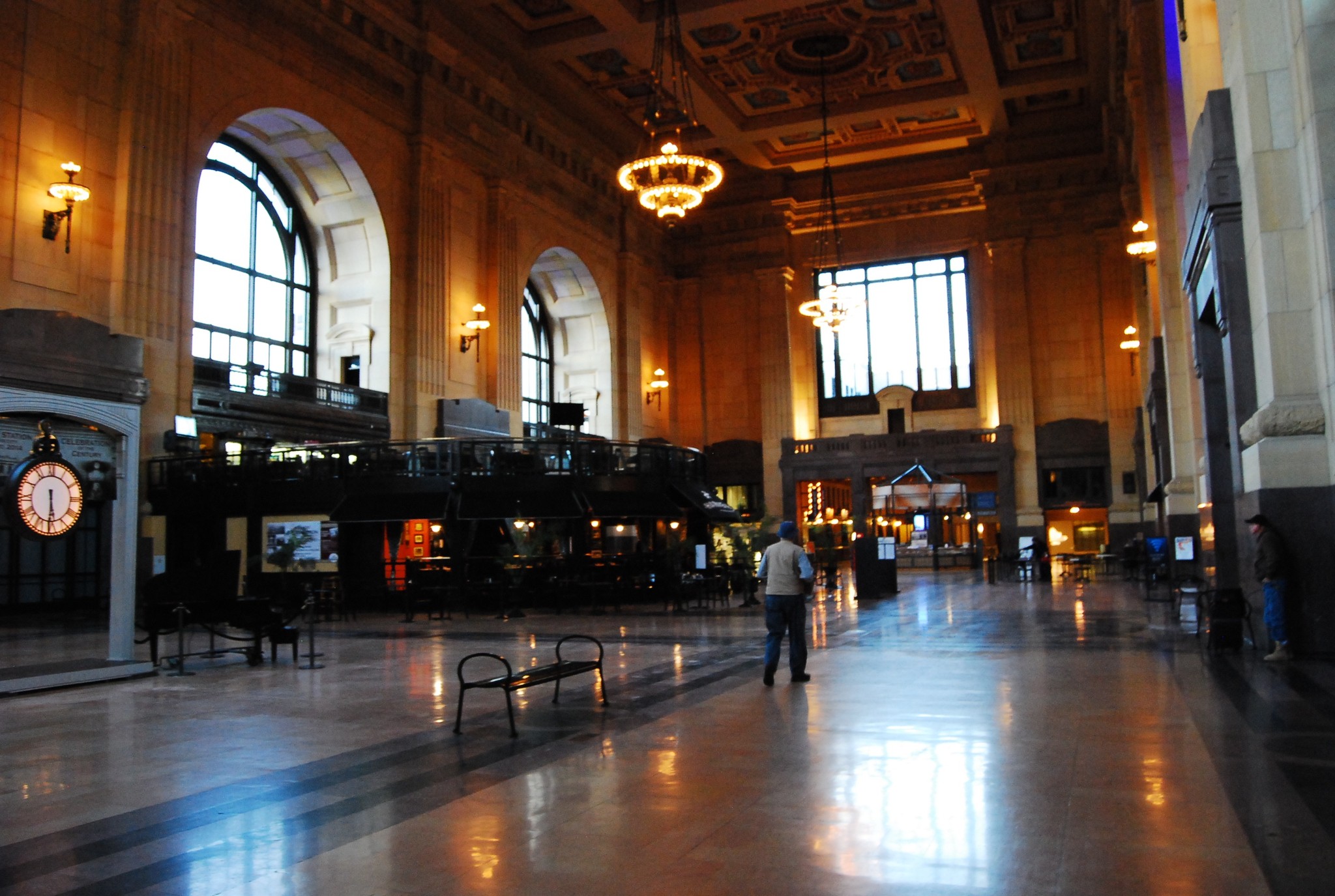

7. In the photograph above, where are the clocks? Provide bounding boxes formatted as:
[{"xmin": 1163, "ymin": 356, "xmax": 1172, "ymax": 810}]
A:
[{"xmin": 3, "ymin": 414, "xmax": 89, "ymax": 545}]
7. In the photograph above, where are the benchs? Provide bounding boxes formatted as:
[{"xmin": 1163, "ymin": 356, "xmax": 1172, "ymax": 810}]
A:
[{"xmin": 454, "ymin": 634, "xmax": 611, "ymax": 738}]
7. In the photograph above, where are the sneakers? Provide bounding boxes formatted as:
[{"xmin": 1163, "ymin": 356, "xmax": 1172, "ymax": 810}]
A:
[
  {"xmin": 763, "ymin": 662, "xmax": 777, "ymax": 687},
  {"xmin": 791, "ymin": 673, "xmax": 810, "ymax": 682}
]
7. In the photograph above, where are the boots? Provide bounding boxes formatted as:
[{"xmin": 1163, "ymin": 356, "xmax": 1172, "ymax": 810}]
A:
[{"xmin": 1264, "ymin": 638, "xmax": 1297, "ymax": 661}]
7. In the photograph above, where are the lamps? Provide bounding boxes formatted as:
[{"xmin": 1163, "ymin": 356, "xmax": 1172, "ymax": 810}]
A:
[
  {"xmin": 41, "ymin": 159, "xmax": 91, "ymax": 252},
  {"xmin": 795, "ymin": 51, "xmax": 858, "ymax": 334},
  {"xmin": 1128, "ymin": 219, "xmax": 1157, "ymax": 264},
  {"xmin": 612, "ymin": 3, "xmax": 727, "ymax": 233},
  {"xmin": 460, "ymin": 301, "xmax": 490, "ymax": 361},
  {"xmin": 646, "ymin": 367, "xmax": 669, "ymax": 409},
  {"xmin": 1119, "ymin": 324, "xmax": 1140, "ymax": 376}
]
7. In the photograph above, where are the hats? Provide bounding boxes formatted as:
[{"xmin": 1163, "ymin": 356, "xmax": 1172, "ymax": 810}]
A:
[
  {"xmin": 1244, "ymin": 514, "xmax": 1267, "ymax": 526},
  {"xmin": 776, "ymin": 521, "xmax": 797, "ymax": 538}
]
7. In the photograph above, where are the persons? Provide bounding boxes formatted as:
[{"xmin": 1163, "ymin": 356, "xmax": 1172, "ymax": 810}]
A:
[
  {"xmin": 1019, "ymin": 537, "xmax": 1051, "ymax": 582},
  {"xmin": 1245, "ymin": 513, "xmax": 1293, "ymax": 663},
  {"xmin": 755, "ymin": 521, "xmax": 815, "ymax": 686}
]
